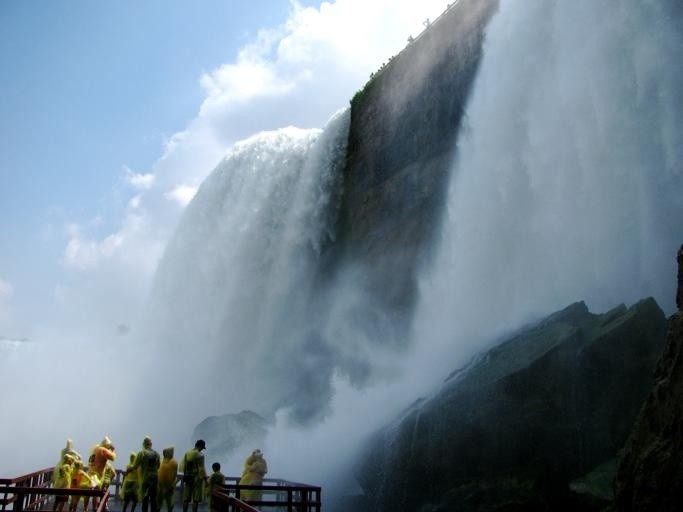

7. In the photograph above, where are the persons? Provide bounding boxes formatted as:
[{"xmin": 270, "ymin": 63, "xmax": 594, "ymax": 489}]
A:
[{"xmin": 52, "ymin": 436, "xmax": 267, "ymax": 512}]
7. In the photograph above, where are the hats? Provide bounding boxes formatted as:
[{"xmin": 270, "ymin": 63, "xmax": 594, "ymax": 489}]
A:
[{"xmin": 195, "ymin": 439, "xmax": 207, "ymax": 449}]
